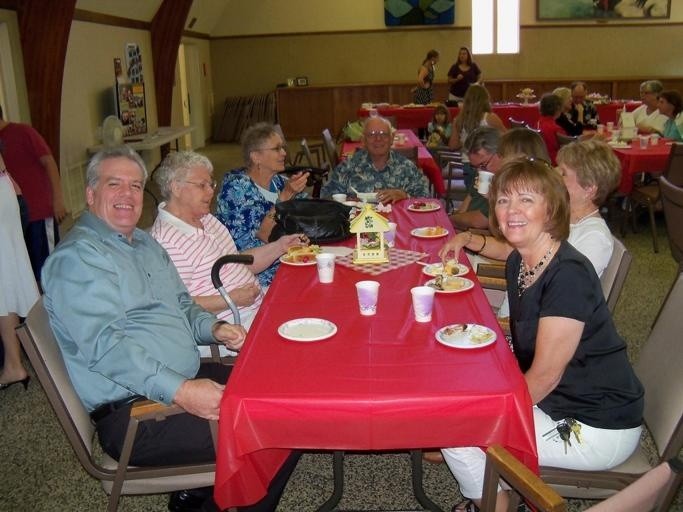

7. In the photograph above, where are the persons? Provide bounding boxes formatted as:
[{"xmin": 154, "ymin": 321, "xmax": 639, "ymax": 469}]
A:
[
  {"xmin": 320, "ymin": 116, "xmax": 430, "ymax": 205},
  {"xmin": 0, "ymin": 102, "xmax": 69, "ymax": 267},
  {"xmin": 213, "ymin": 121, "xmax": 314, "ymax": 295},
  {"xmin": 148, "ymin": 148, "xmax": 311, "ymax": 361},
  {"xmin": 0, "ymin": 151, "xmax": 44, "ymax": 392},
  {"xmin": 423, "ymin": 142, "xmax": 621, "ymax": 461},
  {"xmin": 41, "ymin": 144, "xmax": 244, "ymax": 512},
  {"xmin": 440, "ymin": 158, "xmax": 646, "ymax": 512}
]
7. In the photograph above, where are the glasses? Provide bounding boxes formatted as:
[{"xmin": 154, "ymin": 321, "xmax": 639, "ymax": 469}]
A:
[
  {"xmin": 471, "ymin": 151, "xmax": 498, "ymax": 171},
  {"xmin": 251, "ymin": 144, "xmax": 287, "ymax": 152},
  {"xmin": 174, "ymin": 179, "xmax": 217, "ymax": 192},
  {"xmin": 365, "ymin": 130, "xmax": 391, "ymax": 137},
  {"xmin": 525, "ymin": 156, "xmax": 551, "ymax": 168}
]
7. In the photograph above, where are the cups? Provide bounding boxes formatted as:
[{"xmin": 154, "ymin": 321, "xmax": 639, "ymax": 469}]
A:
[
  {"xmin": 596, "ymin": 125, "xmax": 604, "ymax": 135},
  {"xmin": 332, "ymin": 194, "xmax": 346, "ymax": 203},
  {"xmin": 397, "ymin": 132, "xmax": 405, "ymax": 146},
  {"xmin": 476, "ymin": 171, "xmax": 495, "ymax": 194},
  {"xmin": 380, "ymin": 223, "xmax": 396, "ymax": 247},
  {"xmin": 606, "ymin": 122, "xmax": 613, "ymax": 131},
  {"xmin": 313, "ymin": 253, "xmax": 335, "ymax": 283},
  {"xmin": 611, "ymin": 131, "xmax": 622, "ymax": 145},
  {"xmin": 650, "ymin": 134, "xmax": 658, "ymax": 146},
  {"xmin": 639, "ymin": 137, "xmax": 649, "ymax": 150},
  {"xmin": 409, "ymin": 285, "xmax": 435, "ymax": 322},
  {"xmin": 355, "ymin": 280, "xmax": 381, "ymax": 315}
]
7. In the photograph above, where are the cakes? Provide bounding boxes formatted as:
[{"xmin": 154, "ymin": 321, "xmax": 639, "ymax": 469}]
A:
[{"xmin": 287, "ymin": 246, "xmax": 316, "ymax": 262}]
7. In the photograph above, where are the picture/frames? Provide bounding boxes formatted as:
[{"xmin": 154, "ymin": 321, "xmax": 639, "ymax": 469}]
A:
[
  {"xmin": 534, "ymin": 0, "xmax": 672, "ymax": 21},
  {"xmin": 296, "ymin": 76, "xmax": 308, "ymax": 87}
]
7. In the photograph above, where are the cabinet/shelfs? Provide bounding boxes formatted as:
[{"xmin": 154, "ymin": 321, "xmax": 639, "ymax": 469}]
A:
[{"xmin": 274, "ymin": 73, "xmax": 683, "ymax": 154}]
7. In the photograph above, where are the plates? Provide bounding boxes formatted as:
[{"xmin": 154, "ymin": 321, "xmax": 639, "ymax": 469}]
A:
[
  {"xmin": 355, "ymin": 193, "xmax": 381, "ymax": 202},
  {"xmin": 425, "ymin": 277, "xmax": 475, "ymax": 293},
  {"xmin": 606, "ymin": 144, "xmax": 631, "ymax": 150},
  {"xmin": 276, "ymin": 316, "xmax": 338, "ymax": 342},
  {"xmin": 341, "ymin": 201, "xmax": 355, "ymax": 207},
  {"xmin": 407, "ymin": 204, "xmax": 441, "ymax": 212},
  {"xmin": 437, "ymin": 323, "xmax": 496, "ymax": 349},
  {"xmin": 411, "ymin": 227, "xmax": 449, "ymax": 238},
  {"xmin": 422, "ymin": 262, "xmax": 470, "ymax": 278},
  {"xmin": 278, "ymin": 254, "xmax": 318, "ymax": 265}
]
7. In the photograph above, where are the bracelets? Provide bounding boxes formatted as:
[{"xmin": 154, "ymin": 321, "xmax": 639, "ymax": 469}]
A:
[
  {"xmin": 266, "ymin": 213, "xmax": 275, "ymax": 221},
  {"xmin": 465, "ymin": 229, "xmax": 472, "ymax": 247},
  {"xmin": 479, "ymin": 234, "xmax": 486, "ymax": 255}
]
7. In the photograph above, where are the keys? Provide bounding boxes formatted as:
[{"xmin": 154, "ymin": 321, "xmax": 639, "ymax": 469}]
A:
[{"xmin": 543, "ymin": 416, "xmax": 584, "ymax": 455}]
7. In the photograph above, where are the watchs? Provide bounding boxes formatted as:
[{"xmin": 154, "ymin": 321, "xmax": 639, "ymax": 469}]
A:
[{"xmin": 407, "ymin": 192, "xmax": 412, "ymax": 200}]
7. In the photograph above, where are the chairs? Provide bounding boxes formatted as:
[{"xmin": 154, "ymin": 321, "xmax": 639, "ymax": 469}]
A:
[
  {"xmin": 506, "ymin": 252, "xmax": 682, "ymax": 511},
  {"xmin": 645, "ymin": 174, "xmax": 682, "ymax": 332},
  {"xmin": 14, "ymin": 290, "xmax": 242, "ymax": 511},
  {"xmin": 469, "ymin": 444, "xmax": 683, "ymax": 512},
  {"xmin": 430, "ymin": 144, "xmax": 463, "ymax": 211},
  {"xmin": 291, "ymin": 129, "xmax": 341, "ymax": 186},
  {"xmin": 474, "ymin": 231, "xmax": 630, "ymax": 344},
  {"xmin": 631, "ymin": 142, "xmax": 682, "ymax": 251},
  {"xmin": 554, "ymin": 131, "xmax": 577, "ymax": 149}
]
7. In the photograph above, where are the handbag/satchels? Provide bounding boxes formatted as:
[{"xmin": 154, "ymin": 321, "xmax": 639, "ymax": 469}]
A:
[{"xmin": 274, "ymin": 197, "xmax": 353, "ymax": 245}]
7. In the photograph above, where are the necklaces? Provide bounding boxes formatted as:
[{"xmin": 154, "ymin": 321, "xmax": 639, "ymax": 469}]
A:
[{"xmin": 517, "ymin": 244, "xmax": 553, "ymax": 297}]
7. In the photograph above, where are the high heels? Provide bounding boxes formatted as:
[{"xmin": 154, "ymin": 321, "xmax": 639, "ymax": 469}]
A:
[{"xmin": 0, "ymin": 372, "xmax": 30, "ymax": 392}]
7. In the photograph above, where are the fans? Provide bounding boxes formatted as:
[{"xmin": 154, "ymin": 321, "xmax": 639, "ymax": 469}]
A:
[{"xmin": 90, "ymin": 114, "xmax": 126, "ymax": 151}]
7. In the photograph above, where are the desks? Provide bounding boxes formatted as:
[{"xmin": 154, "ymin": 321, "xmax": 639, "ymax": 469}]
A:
[
  {"xmin": 585, "ymin": 129, "xmax": 682, "ymax": 192},
  {"xmin": 86, "ymin": 124, "xmax": 190, "ymax": 180},
  {"xmin": 358, "ymin": 100, "xmax": 643, "ymax": 134}
]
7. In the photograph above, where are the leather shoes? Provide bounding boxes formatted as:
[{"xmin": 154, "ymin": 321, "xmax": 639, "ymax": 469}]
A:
[{"xmin": 167, "ymin": 484, "xmax": 219, "ymax": 512}]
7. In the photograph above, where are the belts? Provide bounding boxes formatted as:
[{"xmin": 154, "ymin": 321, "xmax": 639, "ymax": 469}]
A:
[{"xmin": 89, "ymin": 393, "xmax": 142, "ymax": 424}]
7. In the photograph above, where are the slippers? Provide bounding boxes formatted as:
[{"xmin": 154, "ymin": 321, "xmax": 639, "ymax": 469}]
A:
[{"xmin": 452, "ymin": 498, "xmax": 526, "ymax": 512}]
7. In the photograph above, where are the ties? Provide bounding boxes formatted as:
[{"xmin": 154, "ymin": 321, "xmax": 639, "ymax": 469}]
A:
[{"xmin": 577, "ymin": 103, "xmax": 584, "ymax": 124}]
